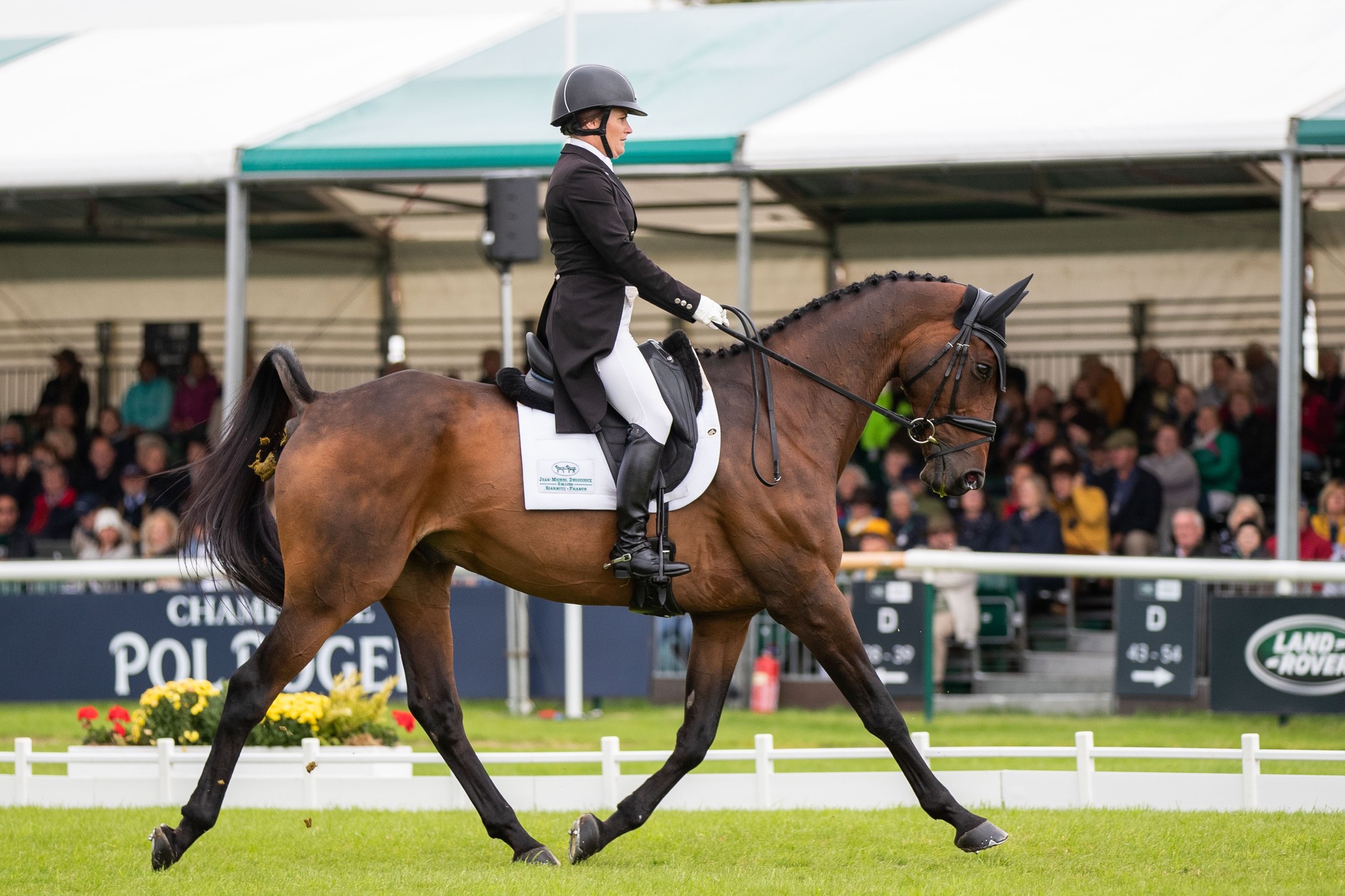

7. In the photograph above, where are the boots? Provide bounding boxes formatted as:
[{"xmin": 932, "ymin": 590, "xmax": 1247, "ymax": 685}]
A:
[{"xmin": 610, "ymin": 423, "xmax": 692, "ymax": 578}]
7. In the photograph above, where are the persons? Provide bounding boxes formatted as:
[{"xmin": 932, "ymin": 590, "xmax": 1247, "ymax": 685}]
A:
[
  {"xmin": 0, "ymin": 340, "xmax": 223, "ymax": 566},
  {"xmin": 474, "ymin": 351, "xmax": 501, "ymax": 384},
  {"xmin": 533, "ymin": 69, "xmax": 728, "ymax": 584},
  {"xmin": 831, "ymin": 343, "xmax": 1345, "ymax": 689}
]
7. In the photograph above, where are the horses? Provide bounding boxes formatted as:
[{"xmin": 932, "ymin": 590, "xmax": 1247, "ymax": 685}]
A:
[{"xmin": 133, "ymin": 273, "xmax": 1036, "ymax": 871}]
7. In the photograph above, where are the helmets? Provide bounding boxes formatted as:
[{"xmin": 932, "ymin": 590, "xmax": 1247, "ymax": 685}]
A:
[{"xmin": 550, "ymin": 65, "xmax": 647, "ymax": 127}]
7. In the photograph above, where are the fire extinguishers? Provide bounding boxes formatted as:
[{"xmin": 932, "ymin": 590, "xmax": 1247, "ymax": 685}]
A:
[{"xmin": 752, "ymin": 646, "xmax": 780, "ymax": 712}]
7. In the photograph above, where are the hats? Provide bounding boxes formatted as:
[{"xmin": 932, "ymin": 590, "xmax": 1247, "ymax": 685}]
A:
[{"xmin": 1106, "ymin": 430, "xmax": 1137, "ymax": 450}]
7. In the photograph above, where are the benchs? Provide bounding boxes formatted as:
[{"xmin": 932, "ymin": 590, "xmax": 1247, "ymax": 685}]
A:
[{"xmin": 947, "ymin": 581, "xmax": 1115, "ymax": 695}]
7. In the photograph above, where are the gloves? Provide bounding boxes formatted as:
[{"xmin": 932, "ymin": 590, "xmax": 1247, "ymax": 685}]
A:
[{"xmin": 692, "ymin": 295, "xmax": 730, "ymax": 331}]
